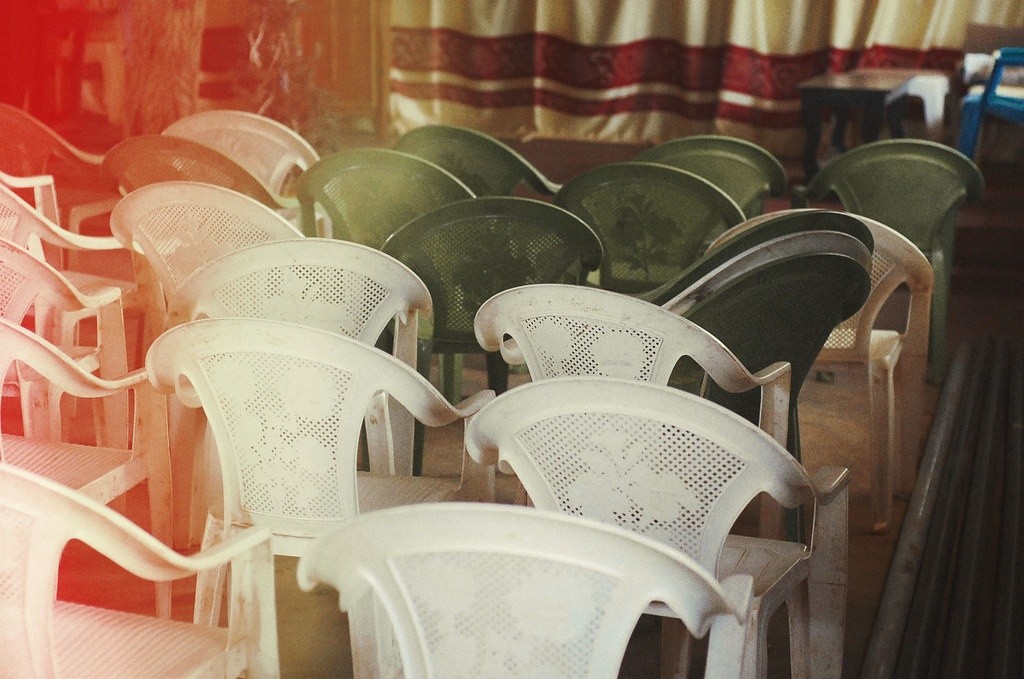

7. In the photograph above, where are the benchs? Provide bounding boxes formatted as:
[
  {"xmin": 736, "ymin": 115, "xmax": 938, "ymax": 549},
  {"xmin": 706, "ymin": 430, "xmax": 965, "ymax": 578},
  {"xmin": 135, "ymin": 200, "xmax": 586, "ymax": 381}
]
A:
[{"xmin": 800, "ymin": 68, "xmax": 951, "ymax": 186}]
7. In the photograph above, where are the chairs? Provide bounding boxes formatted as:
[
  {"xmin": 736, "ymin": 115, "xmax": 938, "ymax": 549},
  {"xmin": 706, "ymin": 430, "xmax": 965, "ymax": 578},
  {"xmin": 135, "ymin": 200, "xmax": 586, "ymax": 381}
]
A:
[{"xmin": 0, "ymin": 47, "xmax": 1024, "ymax": 678}]
